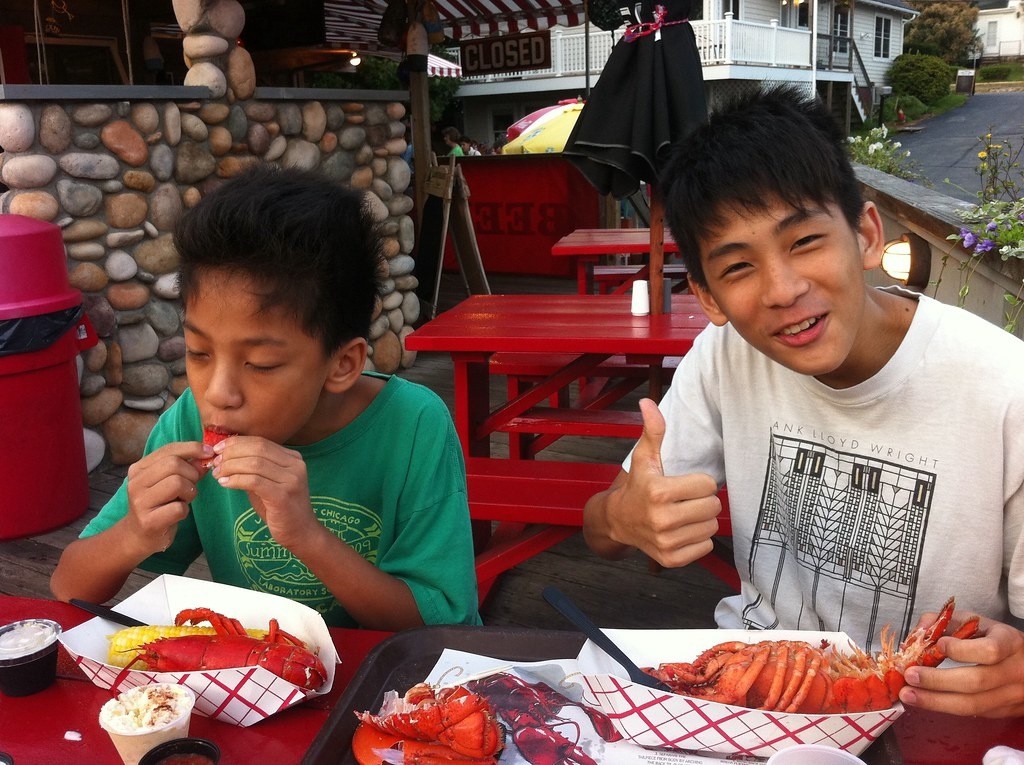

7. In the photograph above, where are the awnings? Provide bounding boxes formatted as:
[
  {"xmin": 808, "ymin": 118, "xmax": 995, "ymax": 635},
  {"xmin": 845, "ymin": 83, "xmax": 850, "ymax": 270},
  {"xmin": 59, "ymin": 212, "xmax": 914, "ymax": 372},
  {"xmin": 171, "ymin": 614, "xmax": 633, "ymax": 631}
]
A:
[
  {"xmin": 325, "ymin": 0, "xmax": 592, "ymax": 43},
  {"xmin": 356, "ymin": 51, "xmax": 463, "ymax": 79}
]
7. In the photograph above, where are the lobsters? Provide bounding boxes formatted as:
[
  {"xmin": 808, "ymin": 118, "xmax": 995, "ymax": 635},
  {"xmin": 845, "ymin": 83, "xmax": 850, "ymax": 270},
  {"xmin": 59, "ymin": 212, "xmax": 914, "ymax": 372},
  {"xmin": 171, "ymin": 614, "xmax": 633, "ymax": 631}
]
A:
[
  {"xmin": 638, "ymin": 598, "xmax": 980, "ymax": 714},
  {"xmin": 113, "ymin": 606, "xmax": 328, "ymax": 688}
]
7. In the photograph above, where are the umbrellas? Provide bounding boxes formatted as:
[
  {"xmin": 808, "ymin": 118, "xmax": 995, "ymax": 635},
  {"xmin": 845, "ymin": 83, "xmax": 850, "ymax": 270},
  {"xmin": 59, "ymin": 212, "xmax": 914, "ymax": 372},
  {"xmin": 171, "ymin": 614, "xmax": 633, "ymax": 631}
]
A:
[{"xmin": 505, "ymin": 0, "xmax": 709, "ymax": 572}]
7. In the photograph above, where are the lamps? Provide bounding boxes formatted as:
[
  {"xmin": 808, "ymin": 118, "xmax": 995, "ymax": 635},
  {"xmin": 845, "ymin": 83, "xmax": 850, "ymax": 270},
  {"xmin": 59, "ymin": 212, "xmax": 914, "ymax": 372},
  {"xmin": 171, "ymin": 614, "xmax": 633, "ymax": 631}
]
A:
[{"xmin": 879, "ymin": 233, "xmax": 931, "ymax": 288}]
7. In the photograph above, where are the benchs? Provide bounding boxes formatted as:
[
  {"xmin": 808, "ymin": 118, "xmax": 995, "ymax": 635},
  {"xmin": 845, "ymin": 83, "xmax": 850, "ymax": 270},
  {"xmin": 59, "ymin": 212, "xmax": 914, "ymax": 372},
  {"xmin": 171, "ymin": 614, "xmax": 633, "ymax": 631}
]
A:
[
  {"xmin": 462, "ymin": 457, "xmax": 733, "ymax": 537},
  {"xmin": 592, "ymin": 266, "xmax": 688, "ymax": 280},
  {"xmin": 490, "ymin": 354, "xmax": 684, "ymax": 380},
  {"xmin": 498, "ymin": 409, "xmax": 645, "ymax": 438}
]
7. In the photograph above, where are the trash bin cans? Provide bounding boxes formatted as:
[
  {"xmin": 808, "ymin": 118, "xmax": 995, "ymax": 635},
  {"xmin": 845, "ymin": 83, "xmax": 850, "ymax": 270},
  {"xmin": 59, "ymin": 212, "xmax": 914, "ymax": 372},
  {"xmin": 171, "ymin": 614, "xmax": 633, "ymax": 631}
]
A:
[{"xmin": 1, "ymin": 214, "xmax": 101, "ymax": 541}]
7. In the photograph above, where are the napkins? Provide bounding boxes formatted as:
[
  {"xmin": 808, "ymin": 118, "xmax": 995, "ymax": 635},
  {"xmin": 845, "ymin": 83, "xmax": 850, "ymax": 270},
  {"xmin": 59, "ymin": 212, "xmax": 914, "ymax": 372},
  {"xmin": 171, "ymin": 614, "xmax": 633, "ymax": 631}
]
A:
[
  {"xmin": 432, "ymin": 665, "xmax": 570, "ymax": 696},
  {"xmin": 982, "ymin": 745, "xmax": 1024, "ymax": 765}
]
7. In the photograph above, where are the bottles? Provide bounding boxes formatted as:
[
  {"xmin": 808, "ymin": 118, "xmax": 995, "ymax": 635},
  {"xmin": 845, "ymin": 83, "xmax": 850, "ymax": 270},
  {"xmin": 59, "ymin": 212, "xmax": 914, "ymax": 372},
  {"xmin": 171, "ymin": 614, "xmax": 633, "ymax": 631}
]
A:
[{"xmin": 631, "ymin": 280, "xmax": 649, "ymax": 317}]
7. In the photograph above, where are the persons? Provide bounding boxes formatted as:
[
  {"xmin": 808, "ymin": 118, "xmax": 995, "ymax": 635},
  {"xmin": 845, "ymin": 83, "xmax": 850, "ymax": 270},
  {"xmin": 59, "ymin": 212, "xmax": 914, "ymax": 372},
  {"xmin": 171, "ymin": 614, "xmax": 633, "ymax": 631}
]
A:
[
  {"xmin": 50, "ymin": 162, "xmax": 485, "ymax": 630},
  {"xmin": 583, "ymin": 86, "xmax": 1024, "ymax": 720},
  {"xmin": 404, "ymin": 123, "xmax": 508, "ymax": 169}
]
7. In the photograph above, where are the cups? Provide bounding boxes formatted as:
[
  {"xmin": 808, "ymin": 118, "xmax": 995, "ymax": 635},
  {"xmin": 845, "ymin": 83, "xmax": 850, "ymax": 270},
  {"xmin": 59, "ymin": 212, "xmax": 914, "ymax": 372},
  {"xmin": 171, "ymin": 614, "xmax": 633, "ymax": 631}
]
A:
[
  {"xmin": 139, "ymin": 738, "xmax": 221, "ymax": 765},
  {"xmin": 99, "ymin": 682, "xmax": 196, "ymax": 765},
  {"xmin": 767, "ymin": 745, "xmax": 867, "ymax": 765},
  {"xmin": 0, "ymin": 618, "xmax": 62, "ymax": 696}
]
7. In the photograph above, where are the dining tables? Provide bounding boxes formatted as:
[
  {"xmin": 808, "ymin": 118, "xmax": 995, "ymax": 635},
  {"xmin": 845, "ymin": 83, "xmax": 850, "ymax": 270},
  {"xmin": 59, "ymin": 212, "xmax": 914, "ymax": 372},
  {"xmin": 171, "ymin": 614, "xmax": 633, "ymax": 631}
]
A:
[{"xmin": 1, "ymin": 596, "xmax": 1024, "ymax": 765}]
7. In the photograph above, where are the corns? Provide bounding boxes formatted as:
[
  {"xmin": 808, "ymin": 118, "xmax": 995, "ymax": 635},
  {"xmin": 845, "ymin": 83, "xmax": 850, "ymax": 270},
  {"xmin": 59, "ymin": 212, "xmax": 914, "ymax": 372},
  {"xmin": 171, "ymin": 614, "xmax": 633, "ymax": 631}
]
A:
[{"xmin": 105, "ymin": 624, "xmax": 308, "ymax": 672}]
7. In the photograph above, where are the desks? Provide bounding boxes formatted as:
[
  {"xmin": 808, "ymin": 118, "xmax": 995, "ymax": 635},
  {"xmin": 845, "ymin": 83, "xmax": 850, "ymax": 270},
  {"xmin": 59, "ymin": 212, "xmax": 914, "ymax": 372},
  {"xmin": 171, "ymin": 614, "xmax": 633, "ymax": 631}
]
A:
[
  {"xmin": 552, "ymin": 229, "xmax": 689, "ymax": 294},
  {"xmin": 406, "ymin": 294, "xmax": 741, "ymax": 608}
]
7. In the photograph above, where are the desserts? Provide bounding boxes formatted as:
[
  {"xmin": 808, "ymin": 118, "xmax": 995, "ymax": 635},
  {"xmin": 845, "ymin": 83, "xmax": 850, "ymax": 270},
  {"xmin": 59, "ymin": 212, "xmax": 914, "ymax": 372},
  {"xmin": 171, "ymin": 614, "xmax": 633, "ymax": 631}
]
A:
[
  {"xmin": 99, "ymin": 683, "xmax": 193, "ymax": 765},
  {"xmin": 0, "ymin": 622, "xmax": 57, "ymax": 660}
]
